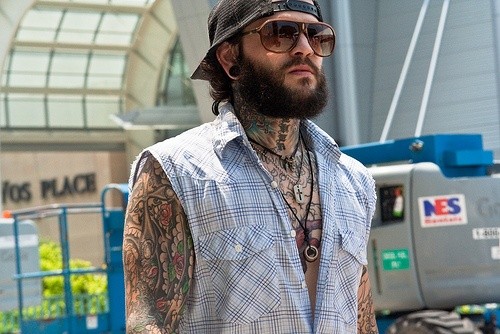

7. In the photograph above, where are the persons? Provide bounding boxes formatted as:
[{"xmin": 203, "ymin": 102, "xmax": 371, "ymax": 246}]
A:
[{"xmin": 121, "ymin": 0, "xmax": 377, "ymax": 334}]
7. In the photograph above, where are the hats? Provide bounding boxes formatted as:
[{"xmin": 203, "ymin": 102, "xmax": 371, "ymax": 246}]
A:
[{"xmin": 190, "ymin": 0, "xmax": 324, "ymax": 81}]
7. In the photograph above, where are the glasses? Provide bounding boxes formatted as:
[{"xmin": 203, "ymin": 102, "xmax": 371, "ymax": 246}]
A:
[{"xmin": 232, "ymin": 18, "xmax": 337, "ymax": 57}]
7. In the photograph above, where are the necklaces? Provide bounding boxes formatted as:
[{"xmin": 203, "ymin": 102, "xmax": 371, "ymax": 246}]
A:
[
  {"xmin": 246, "ymin": 136, "xmax": 301, "ymax": 173},
  {"xmin": 282, "ymin": 129, "xmax": 319, "ymax": 262},
  {"xmin": 253, "ymin": 133, "xmax": 304, "ymax": 206}
]
